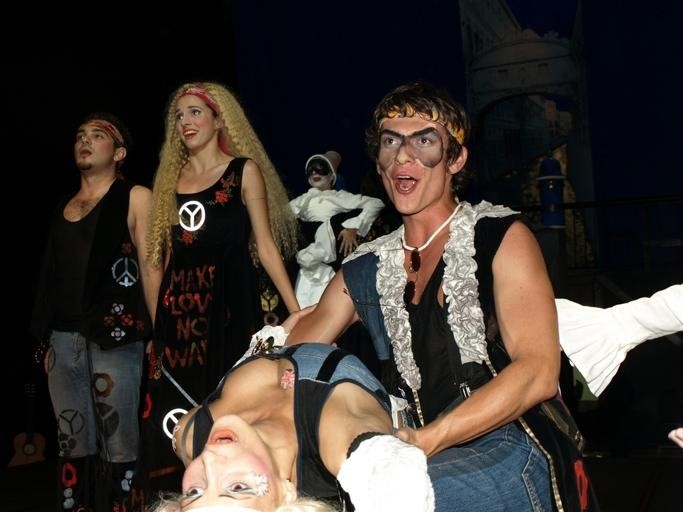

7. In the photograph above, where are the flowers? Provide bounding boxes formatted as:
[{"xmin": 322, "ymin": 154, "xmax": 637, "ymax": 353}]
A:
[{"xmin": 280, "ymin": 370, "xmax": 295, "ymax": 390}]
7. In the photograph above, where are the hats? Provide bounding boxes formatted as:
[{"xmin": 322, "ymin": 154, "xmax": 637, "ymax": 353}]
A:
[{"xmin": 306, "ymin": 151, "xmax": 342, "ymax": 186}]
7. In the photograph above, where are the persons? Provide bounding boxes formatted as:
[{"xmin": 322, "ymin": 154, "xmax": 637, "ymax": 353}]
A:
[{"xmin": 34, "ymin": 76, "xmax": 683, "ymax": 507}]
